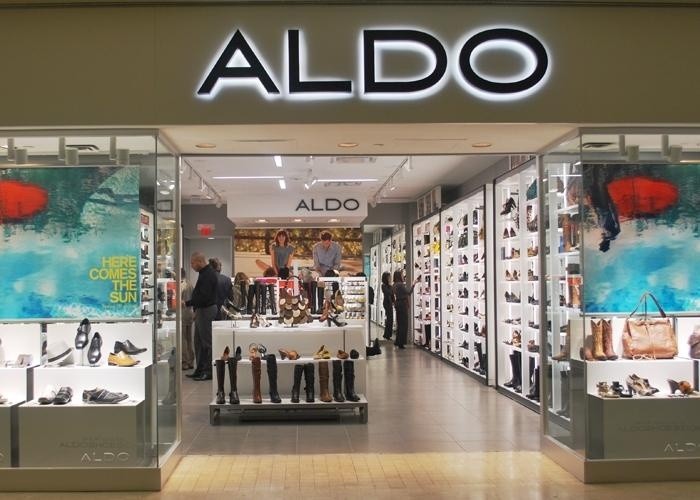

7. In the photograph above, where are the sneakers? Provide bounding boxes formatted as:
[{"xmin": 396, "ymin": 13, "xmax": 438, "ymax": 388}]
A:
[
  {"xmin": 87, "ymin": 389, "xmax": 129, "ymax": 404},
  {"xmin": 54, "ymin": 386, "xmax": 73, "ymax": 403},
  {"xmin": 82, "ymin": 387, "xmax": 100, "ymax": 401},
  {"xmin": 37, "ymin": 386, "xmax": 60, "ymax": 403}
]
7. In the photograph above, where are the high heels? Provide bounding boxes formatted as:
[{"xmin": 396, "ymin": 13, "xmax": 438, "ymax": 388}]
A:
[
  {"xmin": 527, "ymin": 295, "xmax": 538, "ymax": 305},
  {"xmin": 505, "ymin": 270, "xmax": 512, "ymax": 280},
  {"xmin": 221, "ymin": 346, "xmax": 230, "ymax": 361},
  {"xmin": 510, "ymin": 292, "xmax": 520, "ymax": 302},
  {"xmin": 234, "ymin": 346, "xmax": 242, "ymax": 360},
  {"xmin": 505, "ymin": 291, "xmax": 511, "ymax": 302},
  {"xmin": 668, "ymin": 378, "xmax": 694, "ymax": 395},
  {"xmin": 255, "ymin": 314, "xmax": 272, "ymax": 328},
  {"xmin": 625, "ymin": 375, "xmax": 656, "ymax": 397},
  {"xmin": 279, "ymin": 348, "xmax": 300, "ymax": 360},
  {"xmin": 632, "ymin": 373, "xmax": 659, "ymax": 395},
  {"xmin": 326, "ymin": 312, "xmax": 347, "ymax": 327},
  {"xmin": 500, "ymin": 197, "xmax": 516, "ymax": 215}
]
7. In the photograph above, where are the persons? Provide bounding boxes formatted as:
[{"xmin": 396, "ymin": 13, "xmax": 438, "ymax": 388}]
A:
[
  {"xmin": 181, "ymin": 250, "xmax": 225, "ymax": 380},
  {"xmin": 356, "ymin": 272, "xmax": 374, "ymax": 319},
  {"xmin": 312, "ymin": 230, "xmax": 341, "ymax": 276},
  {"xmin": 232, "ymin": 272, "xmax": 251, "ymax": 309},
  {"xmin": 393, "ymin": 270, "xmax": 419, "ymax": 349},
  {"xmin": 382, "ymin": 271, "xmax": 395, "ymax": 340},
  {"xmin": 181, "ymin": 268, "xmax": 194, "ymax": 370},
  {"xmin": 208, "ymin": 256, "xmax": 232, "ymax": 320},
  {"xmin": 271, "ymin": 231, "xmax": 294, "ymax": 275},
  {"xmin": 366, "ymin": 338, "xmax": 382, "ymax": 356}
]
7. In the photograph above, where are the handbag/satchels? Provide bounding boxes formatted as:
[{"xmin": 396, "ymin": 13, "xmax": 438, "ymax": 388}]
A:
[
  {"xmin": 622, "ymin": 292, "xmax": 679, "ymax": 359},
  {"xmin": 278, "ymin": 275, "xmax": 313, "ymax": 325}
]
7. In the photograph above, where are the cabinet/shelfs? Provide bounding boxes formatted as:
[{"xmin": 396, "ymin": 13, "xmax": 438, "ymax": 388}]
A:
[
  {"xmin": 412, "ymin": 209, "xmax": 441, "ymax": 356},
  {"xmin": 319, "ymin": 277, "xmax": 342, "ymax": 301},
  {"xmin": 369, "ymin": 243, "xmax": 378, "ymax": 324},
  {"xmin": 345, "ymin": 277, "xmax": 369, "ymax": 346},
  {"xmin": 1, "ymin": 129, "xmax": 182, "ymax": 491},
  {"xmin": 377, "ymin": 234, "xmax": 392, "ymax": 328},
  {"xmin": 492, "ymin": 158, "xmax": 539, "ymax": 419},
  {"xmin": 440, "ymin": 184, "xmax": 486, "ymax": 384},
  {"xmin": 537, "ymin": 126, "xmax": 700, "ymax": 484},
  {"xmin": 209, "ymin": 316, "xmax": 368, "ymax": 423},
  {"xmin": 390, "ymin": 226, "xmax": 406, "ymax": 336}
]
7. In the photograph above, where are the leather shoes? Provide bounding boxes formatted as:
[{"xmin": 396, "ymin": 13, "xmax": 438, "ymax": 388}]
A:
[
  {"xmin": 185, "ymin": 369, "xmax": 199, "ymax": 378},
  {"xmin": 250, "ymin": 313, "xmax": 259, "ymax": 328},
  {"xmin": 114, "ymin": 340, "xmax": 147, "ymax": 355},
  {"xmin": 87, "ymin": 332, "xmax": 103, "ymax": 364},
  {"xmin": 75, "ymin": 318, "xmax": 91, "ymax": 349},
  {"xmin": 107, "ymin": 351, "xmax": 137, "ymax": 367},
  {"xmin": 193, "ymin": 373, "xmax": 211, "ymax": 381}
]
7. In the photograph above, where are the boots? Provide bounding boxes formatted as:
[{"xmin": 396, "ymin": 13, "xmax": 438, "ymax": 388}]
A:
[
  {"xmin": 228, "ymin": 358, "xmax": 240, "ymax": 404},
  {"xmin": 515, "ymin": 357, "xmax": 535, "ymax": 393},
  {"xmin": 503, "ymin": 350, "xmax": 521, "ymax": 388},
  {"xmin": 525, "ymin": 365, "xmax": 539, "ymax": 400},
  {"xmin": 603, "ymin": 319, "xmax": 618, "ymax": 360},
  {"xmin": 590, "ymin": 319, "xmax": 607, "ymax": 360},
  {"xmin": 331, "ymin": 359, "xmax": 345, "ymax": 402},
  {"xmin": 343, "ymin": 360, "xmax": 360, "ymax": 402},
  {"xmin": 215, "ymin": 359, "xmax": 226, "ymax": 404},
  {"xmin": 266, "ymin": 354, "xmax": 281, "ymax": 403},
  {"xmin": 292, "ymin": 364, "xmax": 304, "ymax": 403},
  {"xmin": 251, "ymin": 357, "xmax": 263, "ymax": 403},
  {"xmin": 304, "ymin": 363, "xmax": 315, "ymax": 402},
  {"xmin": 318, "ymin": 361, "xmax": 333, "ymax": 402}
]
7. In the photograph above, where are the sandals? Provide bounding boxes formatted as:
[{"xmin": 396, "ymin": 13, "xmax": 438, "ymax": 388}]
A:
[
  {"xmin": 313, "ymin": 345, "xmax": 325, "ymax": 359},
  {"xmin": 322, "ymin": 350, "xmax": 329, "ymax": 359},
  {"xmin": 350, "ymin": 349, "xmax": 359, "ymax": 359},
  {"xmin": 503, "ymin": 228, "xmax": 509, "ymax": 238},
  {"xmin": 611, "ymin": 381, "xmax": 632, "ymax": 398},
  {"xmin": 248, "ymin": 343, "xmax": 257, "ymax": 359},
  {"xmin": 336, "ymin": 349, "xmax": 348, "ymax": 359},
  {"xmin": 596, "ymin": 381, "xmax": 620, "ymax": 398},
  {"xmin": 257, "ymin": 343, "xmax": 268, "ymax": 359},
  {"xmin": 510, "ymin": 227, "xmax": 516, "ymax": 237}
]
